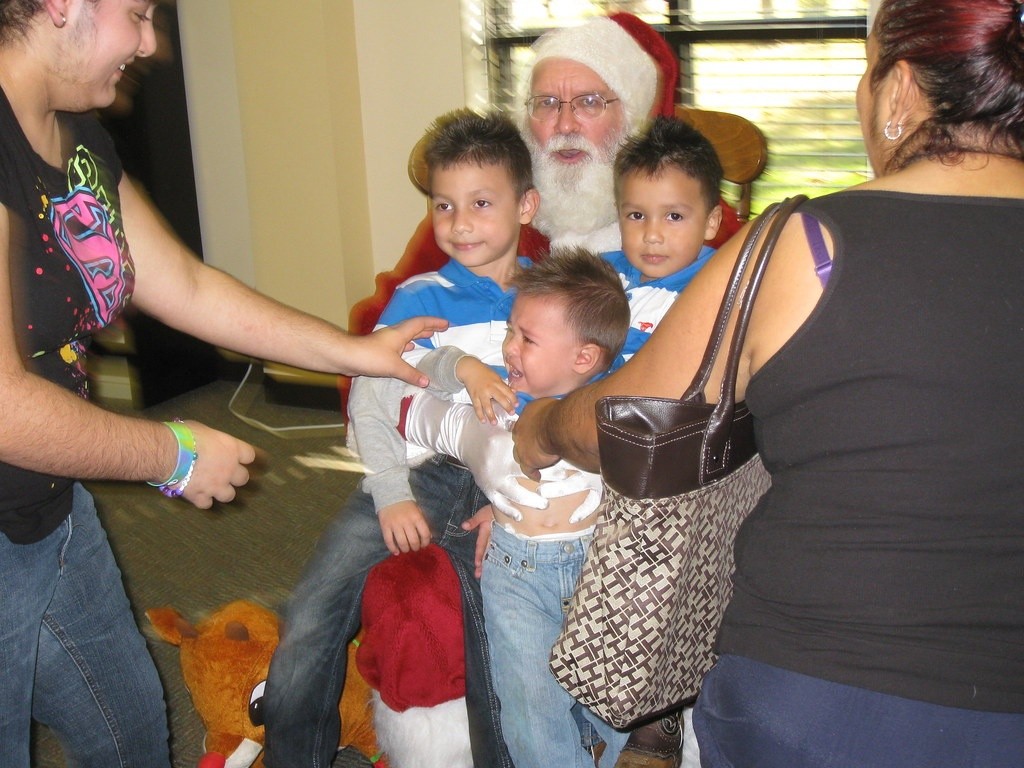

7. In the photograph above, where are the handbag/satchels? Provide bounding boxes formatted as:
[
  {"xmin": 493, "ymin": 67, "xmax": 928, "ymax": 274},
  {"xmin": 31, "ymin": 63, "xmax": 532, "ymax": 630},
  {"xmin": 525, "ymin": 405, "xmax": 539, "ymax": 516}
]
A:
[{"xmin": 549, "ymin": 195, "xmax": 807, "ymax": 729}]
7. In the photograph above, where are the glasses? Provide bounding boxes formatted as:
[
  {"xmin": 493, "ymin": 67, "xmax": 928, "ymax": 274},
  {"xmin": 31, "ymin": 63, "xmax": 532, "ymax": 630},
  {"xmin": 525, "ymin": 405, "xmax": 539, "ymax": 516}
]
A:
[{"xmin": 523, "ymin": 94, "xmax": 618, "ymax": 123}]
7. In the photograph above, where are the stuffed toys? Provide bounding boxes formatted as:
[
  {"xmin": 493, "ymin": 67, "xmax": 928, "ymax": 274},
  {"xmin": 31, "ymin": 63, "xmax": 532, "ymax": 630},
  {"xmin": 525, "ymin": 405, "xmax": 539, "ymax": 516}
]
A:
[{"xmin": 145, "ymin": 593, "xmax": 394, "ymax": 768}]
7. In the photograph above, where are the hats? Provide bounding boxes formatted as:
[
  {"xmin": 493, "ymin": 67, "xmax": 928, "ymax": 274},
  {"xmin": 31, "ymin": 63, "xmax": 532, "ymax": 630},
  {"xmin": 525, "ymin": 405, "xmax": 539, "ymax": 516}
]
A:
[{"xmin": 523, "ymin": 11, "xmax": 677, "ymax": 122}]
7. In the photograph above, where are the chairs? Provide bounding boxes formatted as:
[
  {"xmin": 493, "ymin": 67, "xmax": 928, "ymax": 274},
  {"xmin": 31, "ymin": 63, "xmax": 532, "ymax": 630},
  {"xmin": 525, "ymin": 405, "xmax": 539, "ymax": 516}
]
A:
[{"xmin": 409, "ymin": 105, "xmax": 768, "ymax": 222}]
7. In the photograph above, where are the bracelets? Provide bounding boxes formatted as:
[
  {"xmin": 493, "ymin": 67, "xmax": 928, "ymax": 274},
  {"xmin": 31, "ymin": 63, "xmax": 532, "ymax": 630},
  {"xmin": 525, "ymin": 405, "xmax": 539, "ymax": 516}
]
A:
[{"xmin": 145, "ymin": 418, "xmax": 198, "ymax": 497}]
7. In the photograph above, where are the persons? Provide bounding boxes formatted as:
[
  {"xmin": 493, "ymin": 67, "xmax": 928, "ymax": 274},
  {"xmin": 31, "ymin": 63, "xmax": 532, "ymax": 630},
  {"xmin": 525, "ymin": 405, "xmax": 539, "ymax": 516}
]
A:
[
  {"xmin": 417, "ymin": 244, "xmax": 631, "ymax": 768},
  {"xmin": 264, "ymin": 112, "xmax": 585, "ymax": 768},
  {"xmin": 0, "ymin": 0, "xmax": 449, "ymax": 768},
  {"xmin": 341, "ymin": 11, "xmax": 743, "ymax": 768},
  {"xmin": 514, "ymin": 1, "xmax": 1024, "ymax": 768},
  {"xmin": 597, "ymin": 114, "xmax": 727, "ymax": 383}
]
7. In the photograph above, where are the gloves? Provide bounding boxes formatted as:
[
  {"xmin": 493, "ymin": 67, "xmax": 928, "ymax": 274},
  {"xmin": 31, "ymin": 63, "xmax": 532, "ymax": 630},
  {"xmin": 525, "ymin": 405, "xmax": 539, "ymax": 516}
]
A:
[
  {"xmin": 538, "ymin": 454, "xmax": 603, "ymax": 524},
  {"xmin": 404, "ymin": 391, "xmax": 567, "ymax": 521}
]
